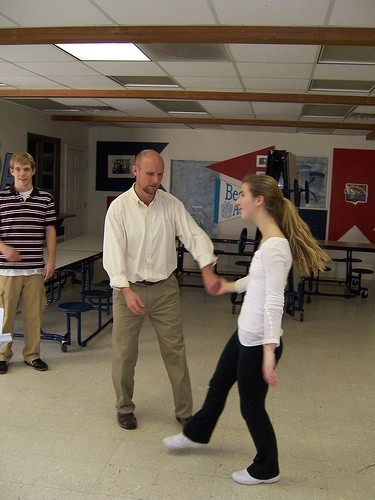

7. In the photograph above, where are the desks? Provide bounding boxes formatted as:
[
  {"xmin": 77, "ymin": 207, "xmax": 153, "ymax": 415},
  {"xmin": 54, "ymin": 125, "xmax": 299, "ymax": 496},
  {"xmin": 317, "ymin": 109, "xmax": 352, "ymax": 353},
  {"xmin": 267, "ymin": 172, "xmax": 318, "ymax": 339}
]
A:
[
  {"xmin": 56, "ymin": 234, "xmax": 113, "ymax": 306},
  {"xmin": 13, "ymin": 248, "xmax": 99, "ymax": 343},
  {"xmin": 304, "ymin": 241, "xmax": 375, "ymax": 298}
]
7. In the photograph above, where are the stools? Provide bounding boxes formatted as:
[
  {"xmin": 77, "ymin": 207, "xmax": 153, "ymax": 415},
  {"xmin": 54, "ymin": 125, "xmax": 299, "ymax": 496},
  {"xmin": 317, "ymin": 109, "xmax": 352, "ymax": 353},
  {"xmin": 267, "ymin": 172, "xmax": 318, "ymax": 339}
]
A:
[
  {"xmin": 57, "ymin": 302, "xmax": 93, "ymax": 345},
  {"xmin": 338, "ymin": 257, "xmax": 362, "ymax": 286},
  {"xmin": 350, "ymin": 269, "xmax": 374, "ymax": 298},
  {"xmin": 235, "ymin": 261, "xmax": 251, "ymax": 275},
  {"xmin": 80, "ymin": 290, "xmax": 109, "ymax": 328},
  {"xmin": 312, "ymin": 267, "xmax": 331, "ymax": 293},
  {"xmin": 100, "ymin": 280, "xmax": 113, "ymax": 316}
]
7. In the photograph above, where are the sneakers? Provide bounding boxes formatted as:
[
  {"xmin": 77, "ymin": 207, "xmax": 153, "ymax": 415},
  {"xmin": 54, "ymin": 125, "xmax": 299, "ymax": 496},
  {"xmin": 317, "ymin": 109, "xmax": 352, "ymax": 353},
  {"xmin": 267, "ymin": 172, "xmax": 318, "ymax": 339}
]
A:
[
  {"xmin": 0, "ymin": 361, "xmax": 7, "ymax": 373},
  {"xmin": 162, "ymin": 432, "xmax": 207, "ymax": 450},
  {"xmin": 231, "ymin": 469, "xmax": 279, "ymax": 484},
  {"xmin": 176, "ymin": 415, "xmax": 192, "ymax": 427},
  {"xmin": 24, "ymin": 358, "xmax": 48, "ymax": 371},
  {"xmin": 117, "ymin": 412, "xmax": 137, "ymax": 428}
]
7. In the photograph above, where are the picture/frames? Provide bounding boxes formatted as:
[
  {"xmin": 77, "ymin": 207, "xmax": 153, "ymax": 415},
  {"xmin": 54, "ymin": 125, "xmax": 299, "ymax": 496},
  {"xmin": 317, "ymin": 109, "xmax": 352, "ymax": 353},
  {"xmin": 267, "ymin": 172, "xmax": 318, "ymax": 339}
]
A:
[{"xmin": 107, "ymin": 155, "xmax": 135, "ymax": 178}]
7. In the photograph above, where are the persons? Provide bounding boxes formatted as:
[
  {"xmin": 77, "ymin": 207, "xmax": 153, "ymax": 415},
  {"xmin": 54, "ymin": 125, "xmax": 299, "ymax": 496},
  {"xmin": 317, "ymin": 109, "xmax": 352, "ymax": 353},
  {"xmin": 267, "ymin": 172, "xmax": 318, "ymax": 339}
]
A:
[
  {"xmin": 103, "ymin": 150, "xmax": 222, "ymax": 430},
  {"xmin": 163, "ymin": 175, "xmax": 331, "ymax": 485},
  {"xmin": 0, "ymin": 151, "xmax": 56, "ymax": 374}
]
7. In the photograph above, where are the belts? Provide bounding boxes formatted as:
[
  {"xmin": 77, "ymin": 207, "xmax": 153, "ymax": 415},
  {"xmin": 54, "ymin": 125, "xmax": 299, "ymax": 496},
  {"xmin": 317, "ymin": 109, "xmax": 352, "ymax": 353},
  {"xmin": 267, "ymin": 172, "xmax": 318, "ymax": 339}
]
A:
[{"xmin": 136, "ymin": 280, "xmax": 162, "ymax": 286}]
